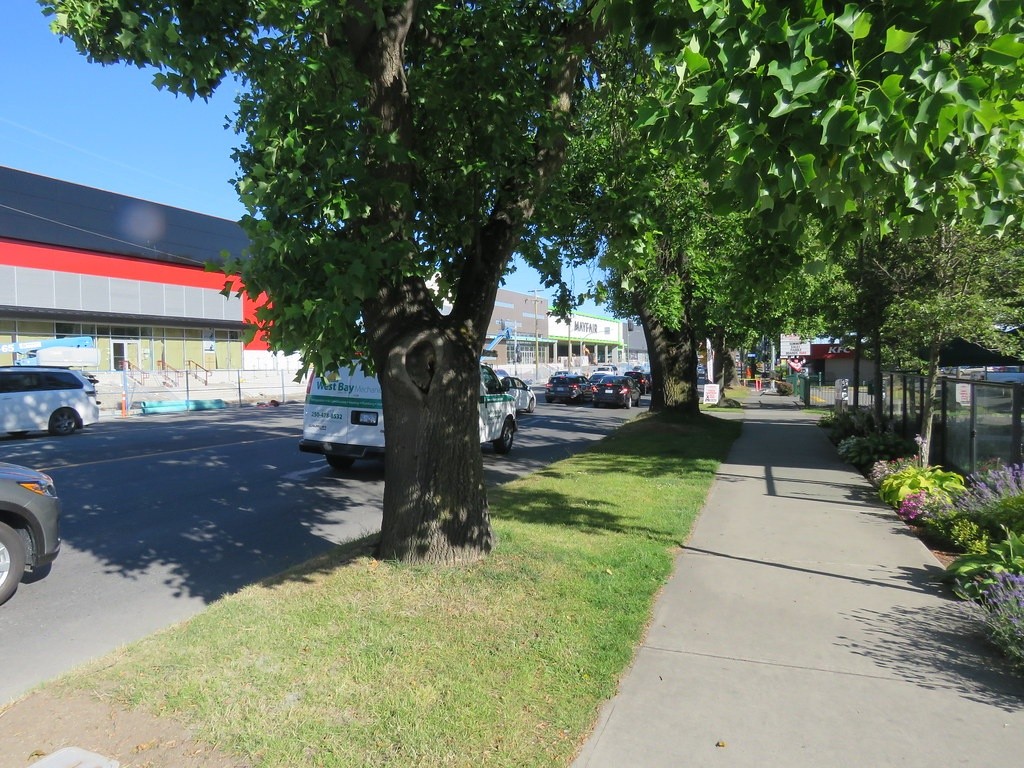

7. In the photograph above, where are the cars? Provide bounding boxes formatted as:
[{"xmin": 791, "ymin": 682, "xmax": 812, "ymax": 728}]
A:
[
  {"xmin": 496, "ymin": 374, "xmax": 537, "ymax": 413},
  {"xmin": 592, "ymin": 375, "xmax": 642, "ymax": 409},
  {"xmin": 0, "ymin": 460, "xmax": 61, "ymax": 605},
  {"xmin": 697, "ymin": 363, "xmax": 707, "ymax": 378},
  {"xmin": 552, "ymin": 362, "xmax": 652, "ymax": 397},
  {"xmin": 544, "ymin": 373, "xmax": 592, "ymax": 405}
]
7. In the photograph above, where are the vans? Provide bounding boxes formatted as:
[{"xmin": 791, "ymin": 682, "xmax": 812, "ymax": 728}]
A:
[{"xmin": 297, "ymin": 329, "xmax": 519, "ymax": 471}]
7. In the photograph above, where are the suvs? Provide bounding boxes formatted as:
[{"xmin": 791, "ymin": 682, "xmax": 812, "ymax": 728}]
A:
[{"xmin": 0, "ymin": 365, "xmax": 100, "ymax": 438}]
